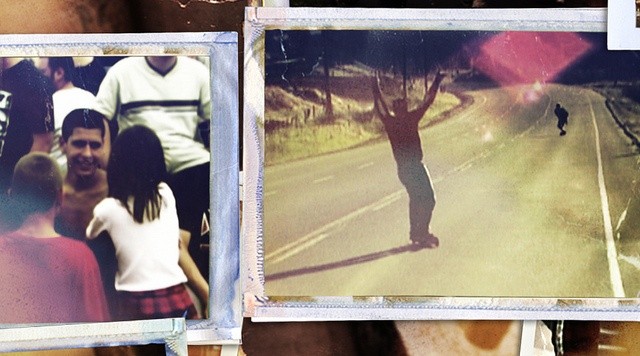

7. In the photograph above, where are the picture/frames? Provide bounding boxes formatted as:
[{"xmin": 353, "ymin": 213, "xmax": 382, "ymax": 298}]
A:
[
  {"xmin": 242, "ymin": 8, "xmax": 640, "ymax": 322},
  {"xmin": 0, "ymin": 30, "xmax": 242, "ymax": 352}
]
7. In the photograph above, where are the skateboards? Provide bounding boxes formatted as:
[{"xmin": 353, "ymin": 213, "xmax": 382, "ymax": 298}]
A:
[{"xmin": 411, "ymin": 236, "xmax": 439, "ymax": 247}]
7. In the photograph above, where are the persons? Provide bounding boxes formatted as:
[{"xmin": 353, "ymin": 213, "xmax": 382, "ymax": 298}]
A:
[
  {"xmin": 54, "ymin": 108, "xmax": 208, "ymax": 318},
  {"xmin": 555, "ymin": 103, "xmax": 568, "ymax": 135},
  {"xmin": 85, "ymin": 125, "xmax": 200, "ymax": 320},
  {"xmin": 39, "ymin": 57, "xmax": 96, "ymax": 172},
  {"xmin": 97, "ymin": 55, "xmax": 210, "ymax": 258},
  {"xmin": 371, "ymin": 68, "xmax": 448, "ymax": 248},
  {"xmin": 1, "ymin": 57, "xmax": 55, "ymax": 196},
  {"xmin": 0, "ymin": 151, "xmax": 112, "ymax": 323}
]
